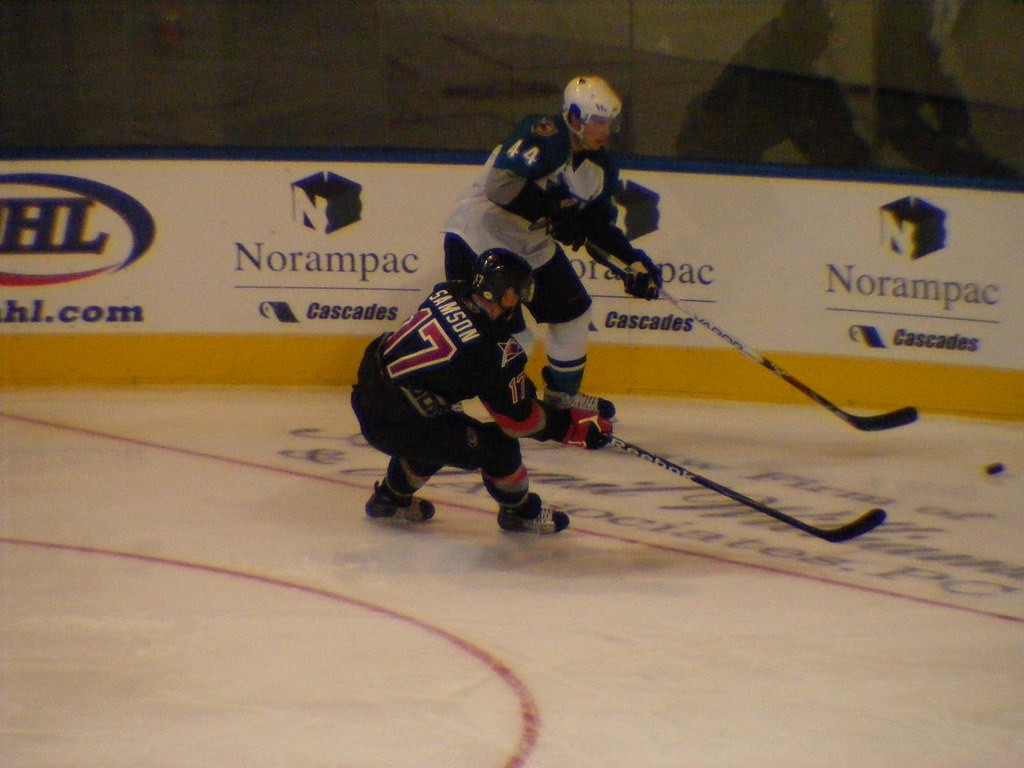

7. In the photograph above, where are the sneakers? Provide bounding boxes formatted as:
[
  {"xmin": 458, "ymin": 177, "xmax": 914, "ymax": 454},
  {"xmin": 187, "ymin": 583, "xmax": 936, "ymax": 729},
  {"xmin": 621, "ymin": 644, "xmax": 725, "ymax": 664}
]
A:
[
  {"xmin": 498, "ymin": 492, "xmax": 569, "ymax": 534},
  {"xmin": 541, "ymin": 365, "xmax": 617, "ymax": 423},
  {"xmin": 365, "ymin": 480, "xmax": 434, "ymax": 525}
]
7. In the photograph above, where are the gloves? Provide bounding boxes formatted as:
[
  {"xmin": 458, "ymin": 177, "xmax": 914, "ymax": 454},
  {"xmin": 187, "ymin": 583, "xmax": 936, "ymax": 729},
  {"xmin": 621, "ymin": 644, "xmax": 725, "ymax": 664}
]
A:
[
  {"xmin": 560, "ymin": 407, "xmax": 613, "ymax": 450},
  {"xmin": 535, "ymin": 180, "xmax": 591, "ymax": 252},
  {"xmin": 618, "ymin": 247, "xmax": 663, "ymax": 301}
]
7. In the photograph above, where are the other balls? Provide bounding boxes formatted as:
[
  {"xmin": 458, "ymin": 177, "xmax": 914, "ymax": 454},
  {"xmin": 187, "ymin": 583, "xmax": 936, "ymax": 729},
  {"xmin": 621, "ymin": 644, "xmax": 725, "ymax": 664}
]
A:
[{"xmin": 987, "ymin": 464, "xmax": 1004, "ymax": 475}]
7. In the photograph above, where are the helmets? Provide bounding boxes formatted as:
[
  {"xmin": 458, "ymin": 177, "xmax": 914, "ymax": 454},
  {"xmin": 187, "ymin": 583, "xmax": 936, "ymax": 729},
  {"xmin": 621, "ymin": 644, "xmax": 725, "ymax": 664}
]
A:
[
  {"xmin": 561, "ymin": 77, "xmax": 623, "ymax": 136},
  {"xmin": 465, "ymin": 248, "xmax": 536, "ymax": 306}
]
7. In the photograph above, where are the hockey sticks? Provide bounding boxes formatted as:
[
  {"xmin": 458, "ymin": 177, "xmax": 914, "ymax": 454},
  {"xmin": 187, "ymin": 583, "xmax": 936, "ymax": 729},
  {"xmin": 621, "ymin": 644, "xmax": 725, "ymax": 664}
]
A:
[
  {"xmin": 547, "ymin": 221, "xmax": 918, "ymax": 433},
  {"xmin": 605, "ymin": 432, "xmax": 888, "ymax": 545}
]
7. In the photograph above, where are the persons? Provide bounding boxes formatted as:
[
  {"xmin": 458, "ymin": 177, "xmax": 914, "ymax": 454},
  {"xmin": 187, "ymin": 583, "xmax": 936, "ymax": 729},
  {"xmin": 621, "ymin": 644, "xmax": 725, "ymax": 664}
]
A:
[
  {"xmin": 440, "ymin": 75, "xmax": 666, "ymax": 424},
  {"xmin": 348, "ymin": 246, "xmax": 613, "ymax": 535}
]
